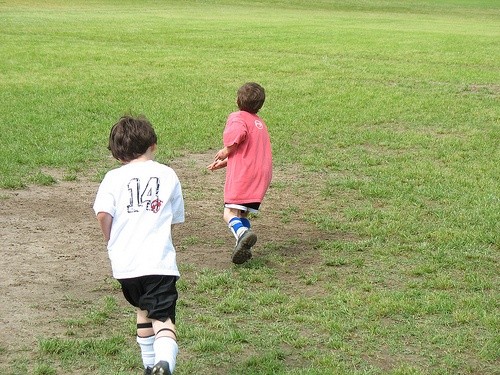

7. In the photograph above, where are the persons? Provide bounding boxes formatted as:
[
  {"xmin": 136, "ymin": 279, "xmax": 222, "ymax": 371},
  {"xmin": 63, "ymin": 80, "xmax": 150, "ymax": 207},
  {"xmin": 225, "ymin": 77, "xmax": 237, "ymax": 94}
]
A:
[
  {"xmin": 206, "ymin": 81, "xmax": 273, "ymax": 265},
  {"xmin": 92, "ymin": 107, "xmax": 186, "ymax": 375}
]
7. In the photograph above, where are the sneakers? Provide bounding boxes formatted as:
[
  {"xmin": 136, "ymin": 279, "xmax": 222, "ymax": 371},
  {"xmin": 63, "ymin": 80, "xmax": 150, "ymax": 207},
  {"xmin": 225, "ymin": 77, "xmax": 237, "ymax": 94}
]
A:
[{"xmin": 232, "ymin": 230, "xmax": 257, "ymax": 265}]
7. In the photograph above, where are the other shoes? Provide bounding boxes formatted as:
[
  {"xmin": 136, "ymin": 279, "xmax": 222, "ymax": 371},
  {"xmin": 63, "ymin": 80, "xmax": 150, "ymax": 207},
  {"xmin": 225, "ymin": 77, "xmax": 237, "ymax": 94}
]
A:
[
  {"xmin": 144, "ymin": 367, "xmax": 152, "ymax": 375},
  {"xmin": 152, "ymin": 361, "xmax": 171, "ymax": 375}
]
